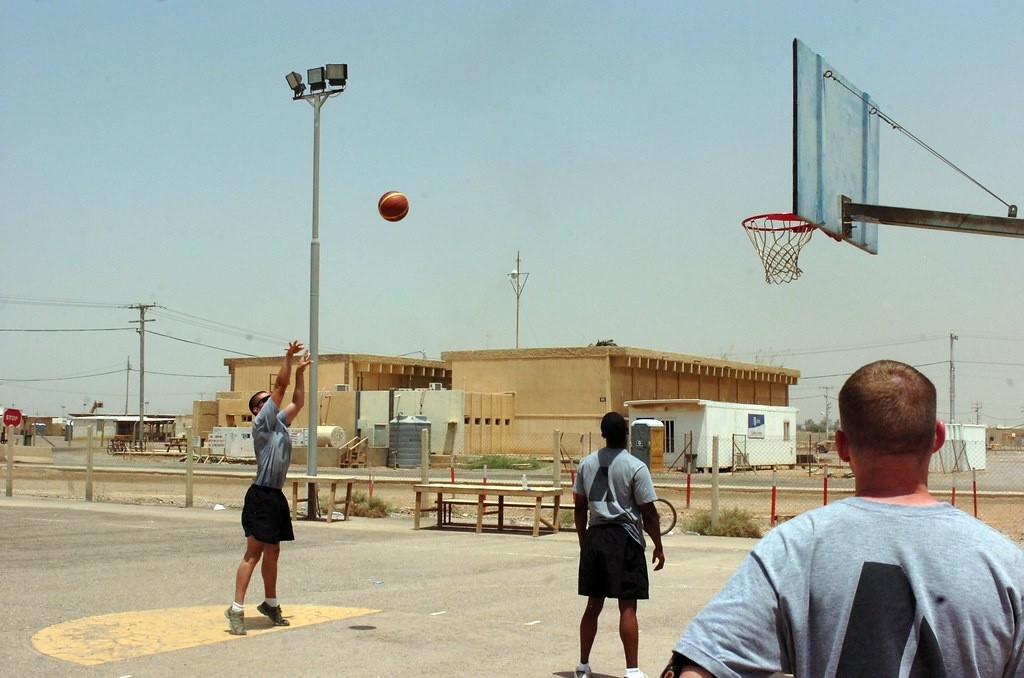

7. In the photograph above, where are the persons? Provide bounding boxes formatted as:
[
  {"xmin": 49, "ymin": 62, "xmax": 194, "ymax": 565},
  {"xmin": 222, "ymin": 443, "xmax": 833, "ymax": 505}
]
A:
[
  {"xmin": 224, "ymin": 340, "xmax": 314, "ymax": 635},
  {"xmin": 672, "ymin": 359, "xmax": 1024, "ymax": 678},
  {"xmin": 571, "ymin": 412, "xmax": 665, "ymax": 678}
]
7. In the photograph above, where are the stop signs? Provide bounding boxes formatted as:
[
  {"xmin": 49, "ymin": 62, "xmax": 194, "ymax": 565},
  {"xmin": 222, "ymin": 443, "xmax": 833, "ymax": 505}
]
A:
[{"xmin": 3, "ymin": 409, "xmax": 22, "ymax": 427}]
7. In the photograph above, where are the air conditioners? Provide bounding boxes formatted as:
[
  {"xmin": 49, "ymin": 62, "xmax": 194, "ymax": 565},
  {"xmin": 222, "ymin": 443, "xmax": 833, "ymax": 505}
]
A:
[
  {"xmin": 429, "ymin": 382, "xmax": 442, "ymax": 391},
  {"xmin": 335, "ymin": 384, "xmax": 350, "ymax": 392}
]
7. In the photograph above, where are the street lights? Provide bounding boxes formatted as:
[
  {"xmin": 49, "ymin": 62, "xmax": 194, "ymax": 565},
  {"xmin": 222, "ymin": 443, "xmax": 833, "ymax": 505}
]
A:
[{"xmin": 286, "ymin": 63, "xmax": 352, "ymax": 521}]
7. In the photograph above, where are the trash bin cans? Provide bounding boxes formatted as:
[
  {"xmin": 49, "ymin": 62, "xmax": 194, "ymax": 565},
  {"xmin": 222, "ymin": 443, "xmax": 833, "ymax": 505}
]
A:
[{"xmin": 685, "ymin": 454, "xmax": 698, "ymax": 472}]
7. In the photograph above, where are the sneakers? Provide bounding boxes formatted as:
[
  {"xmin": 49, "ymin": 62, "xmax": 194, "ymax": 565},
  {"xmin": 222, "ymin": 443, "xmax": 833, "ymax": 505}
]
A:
[
  {"xmin": 574, "ymin": 668, "xmax": 591, "ymax": 678},
  {"xmin": 225, "ymin": 606, "xmax": 246, "ymax": 634},
  {"xmin": 624, "ymin": 673, "xmax": 649, "ymax": 678},
  {"xmin": 257, "ymin": 602, "xmax": 290, "ymax": 626}
]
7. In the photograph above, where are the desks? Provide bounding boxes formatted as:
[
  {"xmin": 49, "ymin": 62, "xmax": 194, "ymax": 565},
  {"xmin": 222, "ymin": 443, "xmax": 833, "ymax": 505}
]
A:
[
  {"xmin": 166, "ymin": 438, "xmax": 187, "ymax": 452},
  {"xmin": 413, "ymin": 483, "xmax": 563, "ymax": 537},
  {"xmin": 283, "ymin": 476, "xmax": 356, "ymax": 522}
]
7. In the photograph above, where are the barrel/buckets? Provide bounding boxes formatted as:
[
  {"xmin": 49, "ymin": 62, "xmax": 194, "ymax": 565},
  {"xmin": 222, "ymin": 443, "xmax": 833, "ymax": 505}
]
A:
[{"xmin": 686, "ymin": 453, "xmax": 697, "ymax": 472}]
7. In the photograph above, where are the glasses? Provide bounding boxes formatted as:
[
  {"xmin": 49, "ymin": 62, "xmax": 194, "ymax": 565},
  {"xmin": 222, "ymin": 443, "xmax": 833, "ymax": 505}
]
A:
[{"xmin": 256, "ymin": 396, "xmax": 270, "ymax": 407}]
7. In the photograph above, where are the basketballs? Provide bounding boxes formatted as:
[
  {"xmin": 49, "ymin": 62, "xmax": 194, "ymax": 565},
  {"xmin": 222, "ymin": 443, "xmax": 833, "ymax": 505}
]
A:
[{"xmin": 378, "ymin": 190, "xmax": 409, "ymax": 223}]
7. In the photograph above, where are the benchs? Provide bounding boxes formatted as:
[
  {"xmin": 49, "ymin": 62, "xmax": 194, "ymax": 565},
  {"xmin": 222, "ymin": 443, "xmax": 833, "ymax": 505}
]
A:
[
  {"xmin": 434, "ymin": 499, "xmax": 589, "ymax": 530},
  {"xmin": 164, "ymin": 444, "xmax": 186, "ymax": 447}
]
